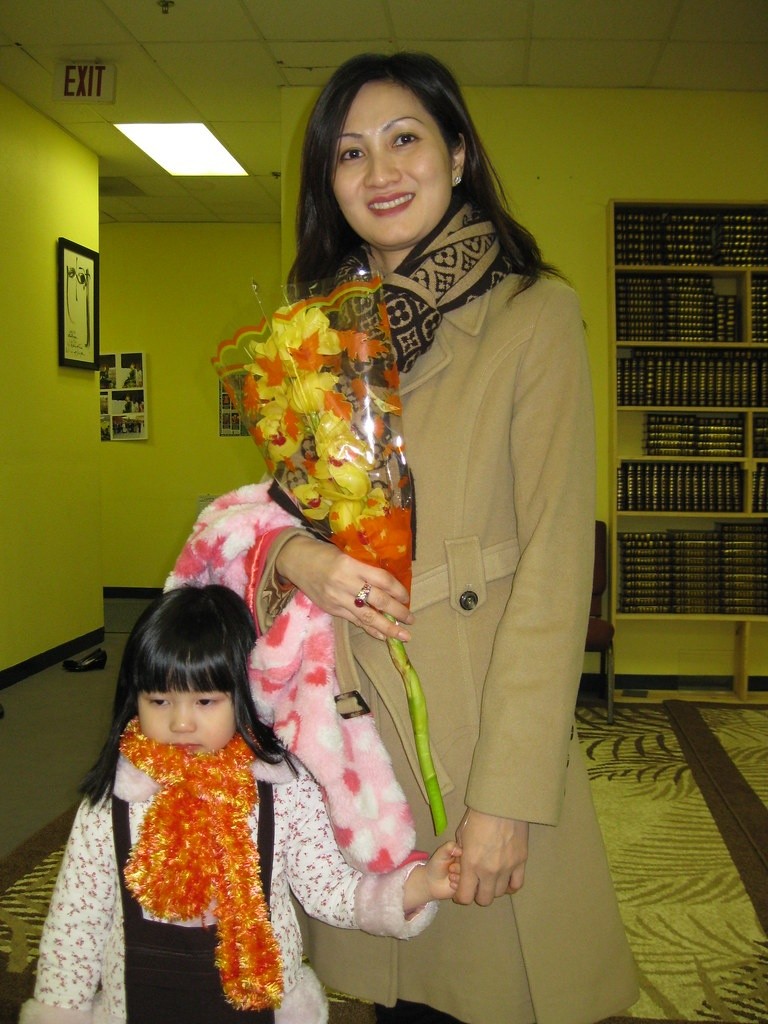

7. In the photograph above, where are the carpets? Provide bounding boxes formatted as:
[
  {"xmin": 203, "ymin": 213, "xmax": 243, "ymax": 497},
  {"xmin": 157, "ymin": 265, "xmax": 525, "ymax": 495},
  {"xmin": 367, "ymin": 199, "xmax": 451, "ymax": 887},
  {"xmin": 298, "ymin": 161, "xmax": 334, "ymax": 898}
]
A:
[{"xmin": 0, "ymin": 702, "xmax": 768, "ymax": 1024}]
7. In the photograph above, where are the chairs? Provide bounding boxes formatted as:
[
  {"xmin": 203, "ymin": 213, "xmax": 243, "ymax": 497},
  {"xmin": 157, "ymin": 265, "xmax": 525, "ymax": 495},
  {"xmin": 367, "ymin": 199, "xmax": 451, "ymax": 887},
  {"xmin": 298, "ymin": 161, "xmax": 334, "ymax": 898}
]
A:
[{"xmin": 574, "ymin": 522, "xmax": 618, "ymax": 707}]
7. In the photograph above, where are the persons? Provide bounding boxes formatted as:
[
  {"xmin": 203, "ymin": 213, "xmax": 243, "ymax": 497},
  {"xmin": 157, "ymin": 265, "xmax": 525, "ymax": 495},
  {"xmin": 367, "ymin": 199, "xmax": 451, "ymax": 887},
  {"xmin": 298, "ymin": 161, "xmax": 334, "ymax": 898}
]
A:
[
  {"xmin": 253, "ymin": 42, "xmax": 648, "ymax": 1024},
  {"xmin": 9, "ymin": 580, "xmax": 463, "ymax": 1024},
  {"xmin": 99, "ymin": 360, "xmax": 144, "ymax": 439}
]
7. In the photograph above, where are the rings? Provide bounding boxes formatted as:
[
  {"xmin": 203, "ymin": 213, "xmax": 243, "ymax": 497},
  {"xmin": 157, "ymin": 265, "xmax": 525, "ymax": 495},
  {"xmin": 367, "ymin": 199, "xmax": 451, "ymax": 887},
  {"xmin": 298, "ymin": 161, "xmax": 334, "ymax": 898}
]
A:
[{"xmin": 355, "ymin": 582, "xmax": 372, "ymax": 608}]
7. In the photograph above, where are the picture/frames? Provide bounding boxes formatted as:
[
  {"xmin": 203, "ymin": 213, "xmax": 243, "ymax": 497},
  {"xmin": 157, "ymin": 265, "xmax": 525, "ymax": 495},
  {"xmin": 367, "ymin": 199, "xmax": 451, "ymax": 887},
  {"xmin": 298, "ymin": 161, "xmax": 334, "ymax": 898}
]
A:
[{"xmin": 57, "ymin": 237, "xmax": 100, "ymax": 370}]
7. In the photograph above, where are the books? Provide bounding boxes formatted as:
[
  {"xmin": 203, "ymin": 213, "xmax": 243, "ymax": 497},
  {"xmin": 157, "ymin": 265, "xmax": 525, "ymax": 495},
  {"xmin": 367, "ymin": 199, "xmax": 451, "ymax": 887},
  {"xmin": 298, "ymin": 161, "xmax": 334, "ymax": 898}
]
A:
[
  {"xmin": 615, "ymin": 274, "xmax": 767, "ymax": 346},
  {"xmin": 616, "ymin": 462, "xmax": 768, "ymax": 514},
  {"xmin": 614, "ymin": 346, "xmax": 767, "ymax": 405},
  {"xmin": 618, "ymin": 517, "xmax": 768, "ymax": 616},
  {"xmin": 639, "ymin": 413, "xmax": 767, "ymax": 459},
  {"xmin": 613, "ymin": 210, "xmax": 768, "ymax": 267}
]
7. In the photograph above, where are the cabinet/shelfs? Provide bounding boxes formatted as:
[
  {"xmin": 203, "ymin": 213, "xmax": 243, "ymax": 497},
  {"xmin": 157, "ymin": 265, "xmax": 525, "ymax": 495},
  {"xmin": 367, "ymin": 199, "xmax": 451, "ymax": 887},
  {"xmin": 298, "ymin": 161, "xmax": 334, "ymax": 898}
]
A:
[{"xmin": 604, "ymin": 198, "xmax": 768, "ymax": 704}]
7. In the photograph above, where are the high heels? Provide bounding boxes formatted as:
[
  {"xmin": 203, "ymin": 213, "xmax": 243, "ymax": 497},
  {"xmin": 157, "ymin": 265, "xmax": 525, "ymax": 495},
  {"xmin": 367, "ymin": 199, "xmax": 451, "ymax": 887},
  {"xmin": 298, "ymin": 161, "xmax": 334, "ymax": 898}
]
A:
[
  {"xmin": 66, "ymin": 651, "xmax": 107, "ymax": 671},
  {"xmin": 62, "ymin": 647, "xmax": 103, "ymax": 667}
]
